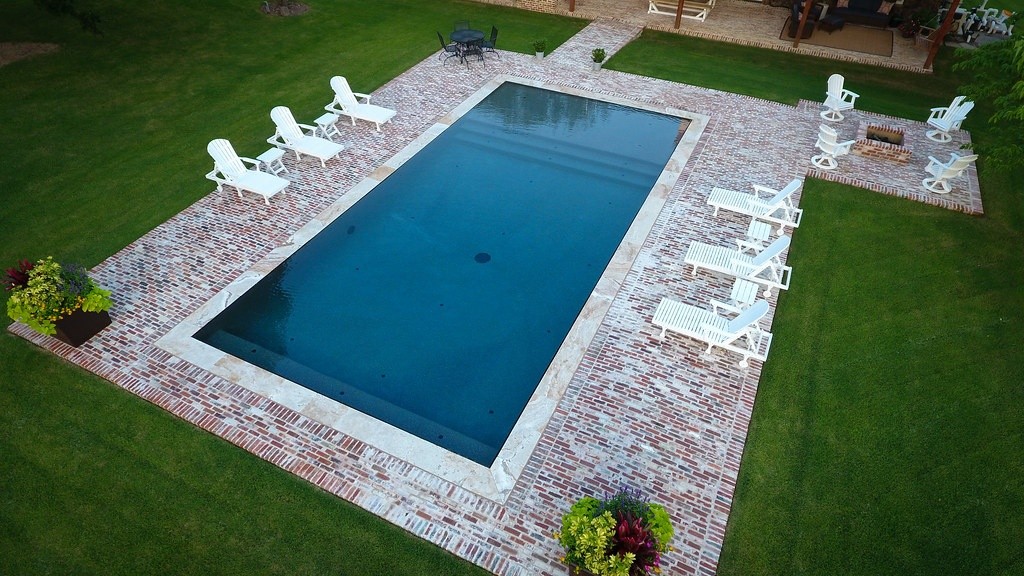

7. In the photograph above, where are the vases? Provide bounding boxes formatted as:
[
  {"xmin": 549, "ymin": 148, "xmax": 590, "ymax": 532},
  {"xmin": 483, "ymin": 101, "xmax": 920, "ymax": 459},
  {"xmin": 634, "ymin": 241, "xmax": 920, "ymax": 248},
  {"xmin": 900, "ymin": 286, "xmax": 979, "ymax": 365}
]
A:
[
  {"xmin": 51, "ymin": 307, "xmax": 112, "ymax": 348},
  {"xmin": 569, "ymin": 562, "xmax": 596, "ymax": 576},
  {"xmin": 903, "ymin": 32, "xmax": 913, "ymax": 38}
]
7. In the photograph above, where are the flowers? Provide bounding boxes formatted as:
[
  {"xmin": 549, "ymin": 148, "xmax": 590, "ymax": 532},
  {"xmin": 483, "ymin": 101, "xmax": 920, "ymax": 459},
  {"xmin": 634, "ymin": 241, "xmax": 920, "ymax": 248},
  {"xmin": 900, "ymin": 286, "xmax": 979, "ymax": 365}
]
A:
[
  {"xmin": 554, "ymin": 488, "xmax": 675, "ymax": 576},
  {"xmin": 898, "ymin": 16, "xmax": 921, "ymax": 34},
  {"xmin": 0, "ymin": 256, "xmax": 114, "ymax": 336}
]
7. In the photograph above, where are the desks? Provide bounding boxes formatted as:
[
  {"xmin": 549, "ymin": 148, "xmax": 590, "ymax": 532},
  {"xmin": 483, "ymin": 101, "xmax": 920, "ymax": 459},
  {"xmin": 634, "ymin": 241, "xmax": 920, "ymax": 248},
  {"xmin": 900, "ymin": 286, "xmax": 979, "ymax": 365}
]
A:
[
  {"xmin": 450, "ymin": 30, "xmax": 486, "ymax": 63},
  {"xmin": 256, "ymin": 147, "xmax": 289, "ymax": 177},
  {"xmin": 723, "ymin": 278, "xmax": 759, "ymax": 315},
  {"xmin": 742, "ymin": 219, "xmax": 772, "ymax": 254},
  {"xmin": 313, "ymin": 113, "xmax": 342, "ymax": 141}
]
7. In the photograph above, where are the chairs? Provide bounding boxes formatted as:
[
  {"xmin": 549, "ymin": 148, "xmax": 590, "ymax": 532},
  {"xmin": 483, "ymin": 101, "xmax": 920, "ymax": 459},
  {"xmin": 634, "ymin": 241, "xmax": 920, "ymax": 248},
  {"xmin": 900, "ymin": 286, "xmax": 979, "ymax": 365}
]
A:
[
  {"xmin": 811, "ymin": 124, "xmax": 856, "ymax": 169},
  {"xmin": 205, "ymin": 138, "xmax": 290, "ymax": 206},
  {"xmin": 436, "ymin": 31, "xmax": 462, "ymax": 66},
  {"xmin": 454, "ymin": 19, "xmax": 470, "ymax": 31},
  {"xmin": 652, "ymin": 296, "xmax": 773, "ymax": 368},
  {"xmin": 820, "ymin": 74, "xmax": 859, "ymax": 122},
  {"xmin": 267, "ymin": 106, "xmax": 345, "ymax": 168},
  {"xmin": 478, "ymin": 26, "xmax": 500, "ymax": 61},
  {"xmin": 707, "ymin": 179, "xmax": 803, "ymax": 235},
  {"xmin": 925, "ymin": 96, "xmax": 974, "ymax": 142},
  {"xmin": 922, "ymin": 153, "xmax": 979, "ymax": 194},
  {"xmin": 324, "ymin": 76, "xmax": 397, "ymax": 131},
  {"xmin": 462, "ymin": 40, "xmax": 485, "ymax": 70},
  {"xmin": 684, "ymin": 235, "xmax": 793, "ymax": 298}
]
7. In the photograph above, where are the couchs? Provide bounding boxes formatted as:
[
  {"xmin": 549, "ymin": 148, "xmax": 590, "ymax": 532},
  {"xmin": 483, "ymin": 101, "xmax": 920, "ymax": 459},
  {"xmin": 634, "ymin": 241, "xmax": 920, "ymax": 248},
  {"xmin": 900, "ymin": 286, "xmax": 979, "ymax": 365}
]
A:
[{"xmin": 829, "ymin": 0, "xmax": 892, "ymax": 29}]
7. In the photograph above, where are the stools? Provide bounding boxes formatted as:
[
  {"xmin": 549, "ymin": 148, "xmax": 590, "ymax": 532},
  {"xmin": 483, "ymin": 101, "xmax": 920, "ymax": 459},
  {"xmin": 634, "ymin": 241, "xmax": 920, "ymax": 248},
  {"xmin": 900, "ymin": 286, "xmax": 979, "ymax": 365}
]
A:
[{"xmin": 818, "ymin": 16, "xmax": 846, "ymax": 35}]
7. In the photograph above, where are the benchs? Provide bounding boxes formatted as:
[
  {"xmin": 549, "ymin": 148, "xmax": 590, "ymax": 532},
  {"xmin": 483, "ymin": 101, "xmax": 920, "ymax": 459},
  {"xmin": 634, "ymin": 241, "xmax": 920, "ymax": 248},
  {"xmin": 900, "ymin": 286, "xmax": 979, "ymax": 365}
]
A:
[{"xmin": 648, "ymin": 0, "xmax": 713, "ymax": 22}]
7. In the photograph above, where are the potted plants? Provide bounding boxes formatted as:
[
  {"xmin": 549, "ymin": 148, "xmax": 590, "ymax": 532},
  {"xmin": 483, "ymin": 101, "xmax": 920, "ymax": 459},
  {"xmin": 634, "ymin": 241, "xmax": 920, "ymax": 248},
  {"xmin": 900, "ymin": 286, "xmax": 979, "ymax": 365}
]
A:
[
  {"xmin": 533, "ymin": 40, "xmax": 546, "ymax": 60},
  {"xmin": 590, "ymin": 48, "xmax": 607, "ymax": 71}
]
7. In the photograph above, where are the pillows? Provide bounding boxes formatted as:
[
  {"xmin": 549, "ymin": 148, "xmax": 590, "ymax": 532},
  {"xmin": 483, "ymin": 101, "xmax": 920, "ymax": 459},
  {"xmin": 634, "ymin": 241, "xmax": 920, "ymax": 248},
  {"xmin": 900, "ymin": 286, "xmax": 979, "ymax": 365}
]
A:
[
  {"xmin": 877, "ymin": 0, "xmax": 894, "ymax": 15},
  {"xmin": 837, "ymin": 0, "xmax": 849, "ymax": 8}
]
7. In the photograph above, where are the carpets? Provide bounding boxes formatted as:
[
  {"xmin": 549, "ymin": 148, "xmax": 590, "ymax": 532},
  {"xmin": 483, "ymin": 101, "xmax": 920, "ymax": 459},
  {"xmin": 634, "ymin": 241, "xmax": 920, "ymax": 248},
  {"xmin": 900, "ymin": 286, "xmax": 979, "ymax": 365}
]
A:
[{"xmin": 780, "ymin": 16, "xmax": 893, "ymax": 56}]
